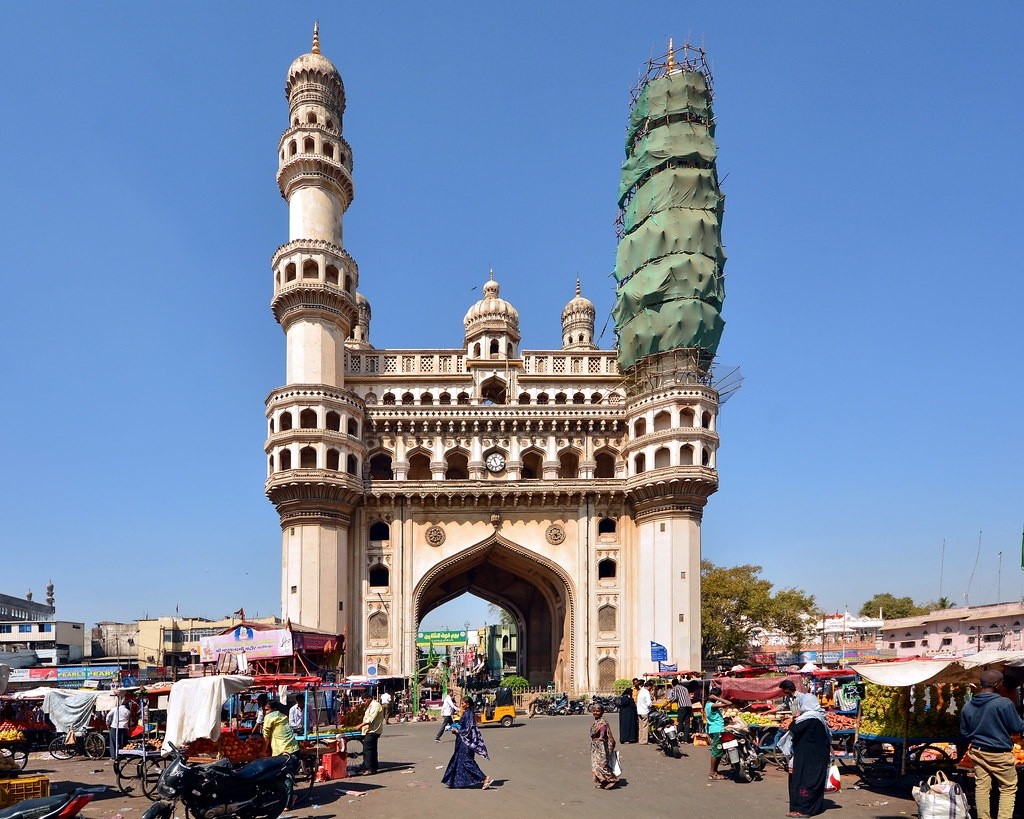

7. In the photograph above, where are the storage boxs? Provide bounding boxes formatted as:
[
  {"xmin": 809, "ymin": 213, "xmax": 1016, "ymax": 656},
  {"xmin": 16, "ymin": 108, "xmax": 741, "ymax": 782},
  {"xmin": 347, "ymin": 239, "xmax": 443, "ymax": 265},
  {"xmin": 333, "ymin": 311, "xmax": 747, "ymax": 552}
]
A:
[
  {"xmin": 691, "ymin": 732, "xmax": 709, "ymax": 746},
  {"xmin": 0, "ymin": 775, "xmax": 51, "ymax": 809},
  {"xmin": 692, "ymin": 701, "xmax": 702, "ymax": 715},
  {"xmin": 321, "ymin": 751, "xmax": 348, "ymax": 779}
]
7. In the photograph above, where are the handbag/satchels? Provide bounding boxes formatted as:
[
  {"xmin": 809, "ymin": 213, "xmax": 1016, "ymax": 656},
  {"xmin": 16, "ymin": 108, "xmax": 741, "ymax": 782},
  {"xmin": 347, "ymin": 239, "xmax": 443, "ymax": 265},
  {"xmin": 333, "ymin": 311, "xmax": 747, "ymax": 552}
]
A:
[
  {"xmin": 912, "ymin": 770, "xmax": 969, "ymax": 819},
  {"xmin": 825, "ymin": 765, "xmax": 841, "ymax": 794},
  {"xmin": 609, "ymin": 751, "xmax": 622, "ymax": 777}
]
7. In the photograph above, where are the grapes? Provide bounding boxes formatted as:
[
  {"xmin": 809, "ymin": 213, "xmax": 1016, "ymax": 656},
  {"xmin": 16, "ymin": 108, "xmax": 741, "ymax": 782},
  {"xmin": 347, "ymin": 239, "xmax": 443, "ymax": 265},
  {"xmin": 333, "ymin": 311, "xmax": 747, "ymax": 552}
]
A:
[{"xmin": 859, "ymin": 683, "xmax": 967, "ymax": 739}]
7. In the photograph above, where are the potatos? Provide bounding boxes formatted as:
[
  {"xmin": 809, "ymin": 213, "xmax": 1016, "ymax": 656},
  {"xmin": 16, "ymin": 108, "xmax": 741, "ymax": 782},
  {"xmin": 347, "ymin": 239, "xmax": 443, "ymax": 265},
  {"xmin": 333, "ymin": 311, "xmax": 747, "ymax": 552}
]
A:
[
  {"xmin": 123, "ymin": 739, "xmax": 163, "ymax": 751},
  {"xmin": 780, "ymin": 712, "xmax": 860, "ymax": 731}
]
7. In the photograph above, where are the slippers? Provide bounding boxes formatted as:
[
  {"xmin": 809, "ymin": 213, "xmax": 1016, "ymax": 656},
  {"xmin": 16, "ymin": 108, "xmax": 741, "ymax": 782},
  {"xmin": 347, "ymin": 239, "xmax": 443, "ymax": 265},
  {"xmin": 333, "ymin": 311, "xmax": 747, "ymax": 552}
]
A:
[
  {"xmin": 482, "ymin": 779, "xmax": 494, "ymax": 790},
  {"xmin": 606, "ymin": 778, "xmax": 619, "ymax": 790}
]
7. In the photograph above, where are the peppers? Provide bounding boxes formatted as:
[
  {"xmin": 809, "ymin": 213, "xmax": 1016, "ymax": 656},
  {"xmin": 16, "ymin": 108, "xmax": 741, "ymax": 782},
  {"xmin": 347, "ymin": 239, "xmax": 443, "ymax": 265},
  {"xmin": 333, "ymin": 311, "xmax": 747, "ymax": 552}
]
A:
[{"xmin": 741, "ymin": 713, "xmax": 773, "ymax": 724}]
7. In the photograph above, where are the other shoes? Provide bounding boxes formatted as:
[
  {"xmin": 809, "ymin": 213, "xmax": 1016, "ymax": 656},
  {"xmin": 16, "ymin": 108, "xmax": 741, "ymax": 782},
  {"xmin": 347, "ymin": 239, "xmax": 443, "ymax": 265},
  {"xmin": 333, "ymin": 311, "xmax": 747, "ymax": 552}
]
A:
[
  {"xmin": 595, "ymin": 784, "xmax": 606, "ymax": 789},
  {"xmin": 786, "ymin": 811, "xmax": 809, "ymax": 818},
  {"xmin": 363, "ymin": 769, "xmax": 377, "ymax": 775}
]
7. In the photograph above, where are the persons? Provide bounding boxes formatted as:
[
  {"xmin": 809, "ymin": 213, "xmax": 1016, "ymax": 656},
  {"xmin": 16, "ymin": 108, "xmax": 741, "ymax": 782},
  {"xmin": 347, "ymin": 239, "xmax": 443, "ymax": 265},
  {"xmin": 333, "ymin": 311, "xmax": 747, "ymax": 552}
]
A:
[
  {"xmin": 289, "ymin": 693, "xmax": 317, "ymax": 782},
  {"xmin": 1, "ymin": 702, "xmax": 47, "ymax": 744},
  {"xmin": 788, "ymin": 693, "xmax": 830, "ymax": 817},
  {"xmin": 380, "ymin": 689, "xmax": 391, "ymax": 724},
  {"xmin": 589, "ymin": 702, "xmax": 620, "ymax": 789},
  {"xmin": 618, "ymin": 678, "xmax": 655, "ymax": 745},
  {"xmin": 435, "ymin": 689, "xmax": 462, "ymax": 743},
  {"xmin": 760, "ymin": 680, "xmax": 803, "ymax": 754},
  {"xmin": 705, "ymin": 687, "xmax": 732, "ymax": 780},
  {"xmin": 105, "ymin": 702, "xmax": 132, "ymax": 761},
  {"xmin": 446, "ymin": 696, "xmax": 495, "ymax": 791},
  {"xmin": 960, "ymin": 670, "xmax": 1023, "ymax": 819},
  {"xmin": 354, "ymin": 694, "xmax": 383, "ymax": 776},
  {"xmin": 666, "ymin": 678, "xmax": 693, "ymax": 744},
  {"xmin": 831, "ymin": 681, "xmax": 858, "ymax": 713},
  {"xmin": 261, "ymin": 699, "xmax": 301, "ymax": 812},
  {"xmin": 250, "ymin": 693, "xmax": 270, "ymax": 736}
]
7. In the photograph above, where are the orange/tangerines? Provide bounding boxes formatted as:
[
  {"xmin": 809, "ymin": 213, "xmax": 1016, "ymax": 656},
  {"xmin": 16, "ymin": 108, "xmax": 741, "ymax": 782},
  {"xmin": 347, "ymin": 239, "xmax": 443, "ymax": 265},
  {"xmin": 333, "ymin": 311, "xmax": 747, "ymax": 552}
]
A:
[{"xmin": 960, "ymin": 733, "xmax": 1024, "ymax": 768}]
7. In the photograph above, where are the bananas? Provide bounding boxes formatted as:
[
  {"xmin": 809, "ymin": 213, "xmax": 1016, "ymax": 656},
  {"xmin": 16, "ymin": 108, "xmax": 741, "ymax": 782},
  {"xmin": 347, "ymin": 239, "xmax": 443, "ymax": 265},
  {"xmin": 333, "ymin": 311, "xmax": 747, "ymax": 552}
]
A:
[{"xmin": 0, "ymin": 729, "xmax": 25, "ymax": 741}]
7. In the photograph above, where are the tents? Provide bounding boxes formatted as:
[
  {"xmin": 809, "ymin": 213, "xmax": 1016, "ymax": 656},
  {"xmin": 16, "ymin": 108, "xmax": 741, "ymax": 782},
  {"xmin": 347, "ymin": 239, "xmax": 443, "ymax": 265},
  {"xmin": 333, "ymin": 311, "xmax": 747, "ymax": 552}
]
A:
[{"xmin": 798, "ymin": 662, "xmax": 832, "ymax": 678}]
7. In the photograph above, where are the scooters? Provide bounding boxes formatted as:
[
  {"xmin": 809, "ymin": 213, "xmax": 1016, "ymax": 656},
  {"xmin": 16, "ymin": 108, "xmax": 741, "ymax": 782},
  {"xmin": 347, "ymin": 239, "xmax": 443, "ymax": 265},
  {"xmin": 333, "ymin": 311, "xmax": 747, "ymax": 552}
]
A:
[{"xmin": 0, "ymin": 785, "xmax": 95, "ymax": 819}]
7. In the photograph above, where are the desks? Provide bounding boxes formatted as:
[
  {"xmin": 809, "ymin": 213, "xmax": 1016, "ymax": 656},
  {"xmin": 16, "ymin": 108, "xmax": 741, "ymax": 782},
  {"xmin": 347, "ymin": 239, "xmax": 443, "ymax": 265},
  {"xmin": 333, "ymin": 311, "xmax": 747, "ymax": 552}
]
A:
[{"xmin": 295, "ymin": 732, "xmax": 361, "ymax": 780}]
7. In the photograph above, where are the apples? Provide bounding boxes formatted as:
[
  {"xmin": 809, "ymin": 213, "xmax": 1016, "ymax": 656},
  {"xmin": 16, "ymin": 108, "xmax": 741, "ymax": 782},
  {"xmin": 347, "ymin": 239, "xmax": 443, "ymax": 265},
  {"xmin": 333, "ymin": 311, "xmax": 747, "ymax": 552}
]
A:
[
  {"xmin": 187, "ymin": 730, "xmax": 264, "ymax": 760},
  {"xmin": 0, "ymin": 718, "xmax": 30, "ymax": 730}
]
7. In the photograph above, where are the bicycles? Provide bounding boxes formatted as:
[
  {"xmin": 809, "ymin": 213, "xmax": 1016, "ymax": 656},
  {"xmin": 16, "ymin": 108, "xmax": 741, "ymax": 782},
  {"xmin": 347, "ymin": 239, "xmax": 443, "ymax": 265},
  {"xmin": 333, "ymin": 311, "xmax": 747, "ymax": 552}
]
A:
[{"xmin": 49, "ymin": 723, "xmax": 106, "ymax": 762}]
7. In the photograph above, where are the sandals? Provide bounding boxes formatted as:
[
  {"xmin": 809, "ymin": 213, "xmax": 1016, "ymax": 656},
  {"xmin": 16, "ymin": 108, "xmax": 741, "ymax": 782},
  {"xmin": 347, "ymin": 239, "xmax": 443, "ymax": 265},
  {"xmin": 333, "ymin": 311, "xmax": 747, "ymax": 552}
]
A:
[{"xmin": 708, "ymin": 772, "xmax": 728, "ymax": 781}]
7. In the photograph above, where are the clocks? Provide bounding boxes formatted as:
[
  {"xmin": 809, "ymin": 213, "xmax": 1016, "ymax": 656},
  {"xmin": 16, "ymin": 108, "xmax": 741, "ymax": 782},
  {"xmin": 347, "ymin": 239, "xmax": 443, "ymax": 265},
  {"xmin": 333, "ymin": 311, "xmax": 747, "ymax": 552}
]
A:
[{"xmin": 487, "ymin": 454, "xmax": 504, "ymax": 471}]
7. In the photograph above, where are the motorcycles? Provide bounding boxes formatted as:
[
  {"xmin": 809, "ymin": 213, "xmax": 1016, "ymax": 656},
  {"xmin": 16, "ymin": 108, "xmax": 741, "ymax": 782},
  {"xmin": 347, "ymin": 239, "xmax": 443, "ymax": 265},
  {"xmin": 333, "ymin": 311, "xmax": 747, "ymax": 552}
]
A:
[
  {"xmin": 643, "ymin": 700, "xmax": 686, "ymax": 759},
  {"xmin": 452, "ymin": 685, "xmax": 516, "ymax": 728},
  {"xmin": 532, "ymin": 690, "xmax": 620, "ymax": 717},
  {"xmin": 716, "ymin": 703, "xmax": 768, "ymax": 783},
  {"xmin": 139, "ymin": 741, "xmax": 298, "ymax": 819}
]
